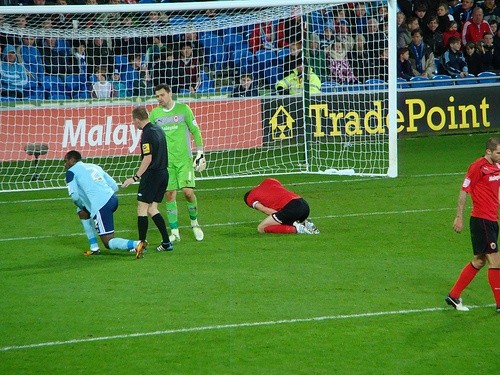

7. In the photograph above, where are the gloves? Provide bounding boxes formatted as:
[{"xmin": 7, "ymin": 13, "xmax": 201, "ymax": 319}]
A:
[
  {"xmin": 192, "ymin": 150, "xmax": 206, "ymax": 172},
  {"xmin": 277, "ymin": 86, "xmax": 287, "ymax": 95}
]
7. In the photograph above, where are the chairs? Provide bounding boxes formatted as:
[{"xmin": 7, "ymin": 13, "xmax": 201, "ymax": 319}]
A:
[{"xmin": 0, "ymin": 0, "xmax": 500, "ymax": 101}]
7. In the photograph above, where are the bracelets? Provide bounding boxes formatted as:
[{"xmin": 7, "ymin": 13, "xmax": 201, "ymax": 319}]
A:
[
  {"xmin": 135, "ymin": 174, "xmax": 139, "ymax": 178},
  {"xmin": 132, "ymin": 177, "xmax": 137, "ymax": 182}
]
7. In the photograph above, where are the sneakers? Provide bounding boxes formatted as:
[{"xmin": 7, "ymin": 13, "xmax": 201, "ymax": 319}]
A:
[
  {"xmin": 84, "ymin": 249, "xmax": 100, "ymax": 255},
  {"xmin": 155, "ymin": 242, "xmax": 173, "ymax": 251},
  {"xmin": 444, "ymin": 294, "xmax": 470, "ymax": 312},
  {"xmin": 160, "ymin": 234, "xmax": 180, "ymax": 242},
  {"xmin": 191, "ymin": 223, "xmax": 203, "ymax": 240},
  {"xmin": 130, "ymin": 248, "xmax": 147, "ymax": 252},
  {"xmin": 135, "ymin": 241, "xmax": 148, "ymax": 258},
  {"xmin": 305, "ymin": 219, "xmax": 320, "ymax": 234},
  {"xmin": 293, "ymin": 221, "xmax": 312, "ymax": 235}
]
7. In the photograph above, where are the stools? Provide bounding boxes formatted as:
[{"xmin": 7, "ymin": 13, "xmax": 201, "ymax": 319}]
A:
[{"xmin": 24, "ymin": 144, "xmax": 48, "ymax": 181}]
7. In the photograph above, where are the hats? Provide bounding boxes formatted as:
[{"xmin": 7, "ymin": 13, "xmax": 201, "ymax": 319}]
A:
[{"xmin": 114, "ymin": 69, "xmax": 119, "ymax": 74}]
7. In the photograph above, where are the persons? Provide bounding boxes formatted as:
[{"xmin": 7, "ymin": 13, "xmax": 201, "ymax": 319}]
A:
[
  {"xmin": 397, "ymin": 0, "xmax": 500, "ymax": 80},
  {"xmin": 444, "ymin": 136, "xmax": 500, "ymax": 313},
  {"xmin": 0, "ymin": 0, "xmax": 389, "ymax": 100},
  {"xmin": 147, "ymin": 83, "xmax": 208, "ymax": 241},
  {"xmin": 120, "ymin": 107, "xmax": 174, "ymax": 252},
  {"xmin": 63, "ymin": 151, "xmax": 148, "ymax": 259},
  {"xmin": 244, "ymin": 177, "xmax": 321, "ymax": 235}
]
